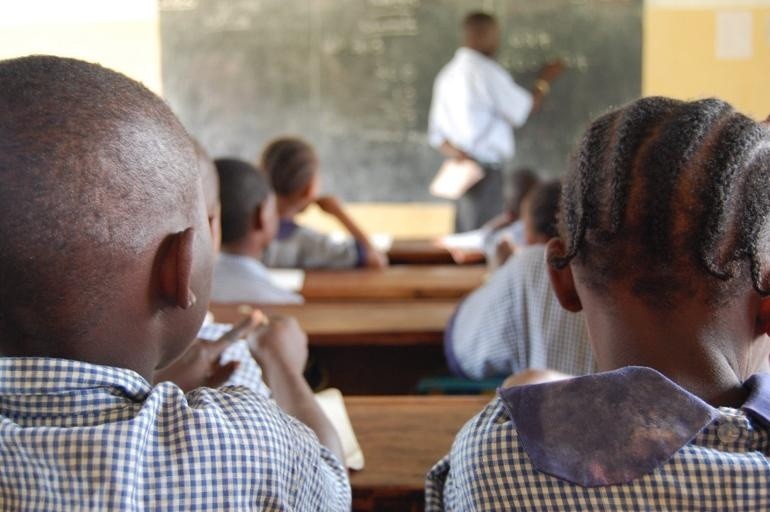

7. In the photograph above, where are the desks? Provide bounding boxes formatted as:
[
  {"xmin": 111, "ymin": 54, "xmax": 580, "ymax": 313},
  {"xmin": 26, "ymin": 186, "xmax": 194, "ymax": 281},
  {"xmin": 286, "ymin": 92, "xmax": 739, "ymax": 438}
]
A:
[
  {"xmin": 208, "ymin": 297, "xmax": 458, "ymax": 350},
  {"xmin": 273, "ymin": 264, "xmax": 488, "ymax": 296},
  {"xmin": 385, "ymin": 239, "xmax": 491, "ymax": 260},
  {"xmin": 316, "ymin": 393, "xmax": 496, "ymax": 493}
]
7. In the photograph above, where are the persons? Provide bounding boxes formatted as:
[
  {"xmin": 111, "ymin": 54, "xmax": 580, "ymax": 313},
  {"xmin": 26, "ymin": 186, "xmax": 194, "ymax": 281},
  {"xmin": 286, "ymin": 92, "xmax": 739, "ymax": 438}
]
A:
[
  {"xmin": 441, "ymin": 235, "xmax": 600, "ymax": 379},
  {"xmin": 426, "ymin": 10, "xmax": 565, "ymax": 236},
  {"xmin": 452, "ymin": 168, "xmax": 546, "ymax": 263},
  {"xmin": 259, "ymin": 136, "xmax": 390, "ymax": 270},
  {"xmin": 0, "ymin": 55, "xmax": 356, "ymax": 512},
  {"xmin": 422, "ymin": 93, "xmax": 770, "ymax": 510},
  {"xmin": 189, "ymin": 134, "xmax": 280, "ymax": 404},
  {"xmin": 485, "ymin": 181, "xmax": 568, "ymax": 270},
  {"xmin": 211, "ymin": 155, "xmax": 307, "ymax": 307}
]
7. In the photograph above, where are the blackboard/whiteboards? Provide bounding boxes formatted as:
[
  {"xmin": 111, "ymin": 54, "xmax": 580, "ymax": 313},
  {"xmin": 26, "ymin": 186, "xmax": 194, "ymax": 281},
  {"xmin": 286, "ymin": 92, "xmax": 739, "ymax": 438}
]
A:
[{"xmin": 158, "ymin": 0, "xmax": 644, "ymax": 203}]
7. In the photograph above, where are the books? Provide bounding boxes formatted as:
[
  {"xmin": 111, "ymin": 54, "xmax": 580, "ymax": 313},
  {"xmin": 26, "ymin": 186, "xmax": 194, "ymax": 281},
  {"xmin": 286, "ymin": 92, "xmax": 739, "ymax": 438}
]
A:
[
  {"xmin": 314, "ymin": 384, "xmax": 366, "ymax": 471},
  {"xmin": 429, "ymin": 157, "xmax": 486, "ymax": 201}
]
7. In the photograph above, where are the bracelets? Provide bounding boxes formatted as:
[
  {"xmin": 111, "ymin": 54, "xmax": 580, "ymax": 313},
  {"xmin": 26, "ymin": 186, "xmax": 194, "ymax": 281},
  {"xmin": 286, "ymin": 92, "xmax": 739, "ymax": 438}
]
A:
[{"xmin": 536, "ymin": 78, "xmax": 552, "ymax": 97}]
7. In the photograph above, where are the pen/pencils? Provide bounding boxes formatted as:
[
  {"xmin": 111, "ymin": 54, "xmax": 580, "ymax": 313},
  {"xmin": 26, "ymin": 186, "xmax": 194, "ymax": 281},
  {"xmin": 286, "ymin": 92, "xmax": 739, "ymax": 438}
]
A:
[{"xmin": 237, "ymin": 304, "xmax": 270, "ymax": 328}]
